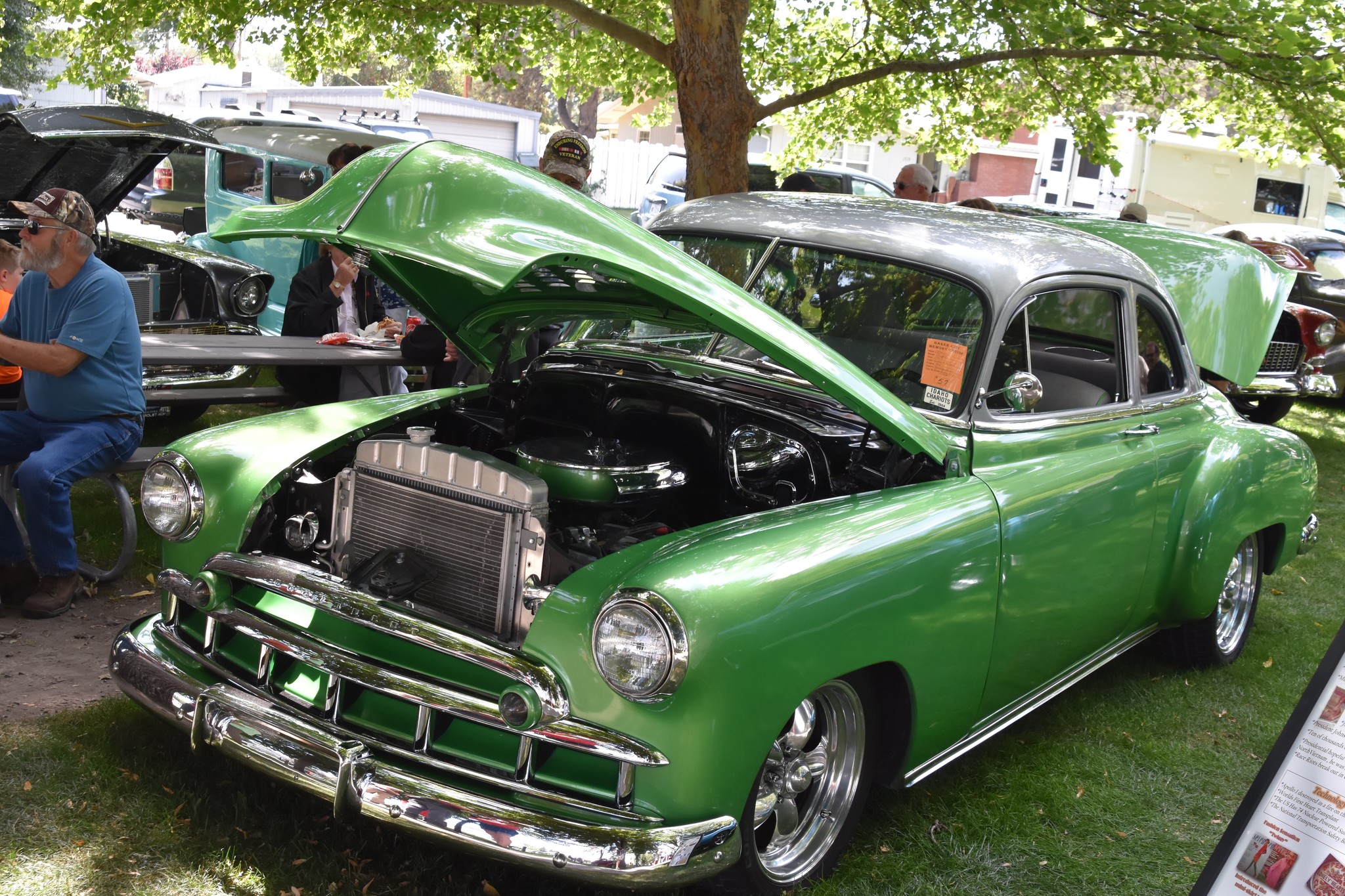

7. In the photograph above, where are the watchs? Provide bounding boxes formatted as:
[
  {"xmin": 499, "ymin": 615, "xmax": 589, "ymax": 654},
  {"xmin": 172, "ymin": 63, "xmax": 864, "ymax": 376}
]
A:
[{"xmin": 331, "ymin": 282, "xmax": 346, "ymax": 291}]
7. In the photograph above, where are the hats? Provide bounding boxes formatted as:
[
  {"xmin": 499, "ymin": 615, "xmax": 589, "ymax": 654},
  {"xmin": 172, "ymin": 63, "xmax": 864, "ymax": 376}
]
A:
[
  {"xmin": 1120, "ymin": 203, "xmax": 1147, "ymax": 224},
  {"xmin": 931, "ymin": 184, "xmax": 940, "ymax": 193},
  {"xmin": 7, "ymin": 188, "xmax": 94, "ymax": 236},
  {"xmin": 782, "ymin": 172, "xmax": 826, "ymax": 192},
  {"xmin": 542, "ymin": 129, "xmax": 591, "ymax": 185}
]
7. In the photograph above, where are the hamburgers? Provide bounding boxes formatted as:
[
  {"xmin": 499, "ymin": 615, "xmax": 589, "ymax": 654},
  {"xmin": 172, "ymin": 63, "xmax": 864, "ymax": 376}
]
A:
[{"xmin": 377, "ymin": 317, "xmax": 394, "ymax": 332}]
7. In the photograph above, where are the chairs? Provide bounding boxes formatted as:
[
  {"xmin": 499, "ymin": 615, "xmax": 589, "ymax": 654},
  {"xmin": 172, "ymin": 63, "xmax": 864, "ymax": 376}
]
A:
[
  {"xmin": 818, "ymin": 334, "xmax": 910, "ymax": 389},
  {"xmin": 1031, "ymin": 368, "xmax": 1109, "ymax": 414}
]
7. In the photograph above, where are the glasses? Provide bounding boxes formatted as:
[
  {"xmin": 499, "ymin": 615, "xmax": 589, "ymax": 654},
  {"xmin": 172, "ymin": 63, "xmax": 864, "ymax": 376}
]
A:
[
  {"xmin": 22, "ymin": 218, "xmax": 70, "ymax": 235},
  {"xmin": 338, "ymin": 296, "xmax": 358, "ymax": 325},
  {"xmin": 543, "ymin": 165, "xmax": 583, "ymax": 191},
  {"xmin": 893, "ymin": 181, "xmax": 922, "ymax": 191},
  {"xmin": 329, "ymin": 166, "xmax": 339, "ymax": 175}
]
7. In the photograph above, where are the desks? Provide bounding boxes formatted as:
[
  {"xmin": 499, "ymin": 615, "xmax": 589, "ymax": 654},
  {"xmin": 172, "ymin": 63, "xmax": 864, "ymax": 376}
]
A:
[{"xmin": 3, "ymin": 331, "xmax": 417, "ymax": 582}]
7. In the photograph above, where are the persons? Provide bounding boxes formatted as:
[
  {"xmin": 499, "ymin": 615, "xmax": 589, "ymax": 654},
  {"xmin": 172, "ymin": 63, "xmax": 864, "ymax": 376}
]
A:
[
  {"xmin": 0, "ymin": 194, "xmax": 147, "ymax": 619},
  {"xmin": 1140, "ymin": 341, "xmax": 1173, "ymax": 392},
  {"xmin": 893, "ymin": 164, "xmax": 1253, "ymax": 393},
  {"xmin": 275, "ymin": 130, "xmax": 592, "ymax": 407}
]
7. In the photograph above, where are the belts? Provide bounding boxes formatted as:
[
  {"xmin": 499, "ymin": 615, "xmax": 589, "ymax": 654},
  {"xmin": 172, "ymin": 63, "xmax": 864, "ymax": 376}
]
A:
[{"xmin": 120, "ymin": 414, "xmax": 140, "ymax": 422}]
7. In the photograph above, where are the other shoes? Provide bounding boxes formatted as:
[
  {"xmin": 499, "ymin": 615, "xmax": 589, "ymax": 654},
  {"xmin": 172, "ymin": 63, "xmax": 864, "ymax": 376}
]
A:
[
  {"xmin": 21, "ymin": 569, "xmax": 83, "ymax": 618},
  {"xmin": 0, "ymin": 559, "xmax": 39, "ymax": 597}
]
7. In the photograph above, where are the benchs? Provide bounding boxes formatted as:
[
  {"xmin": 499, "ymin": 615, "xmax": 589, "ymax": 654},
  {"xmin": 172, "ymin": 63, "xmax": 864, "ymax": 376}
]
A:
[
  {"xmin": 864, "ymin": 325, "xmax": 1117, "ymax": 404},
  {"xmin": 112, "ymin": 444, "xmax": 165, "ymax": 472},
  {"xmin": 145, "ymin": 386, "xmax": 286, "ymax": 405}
]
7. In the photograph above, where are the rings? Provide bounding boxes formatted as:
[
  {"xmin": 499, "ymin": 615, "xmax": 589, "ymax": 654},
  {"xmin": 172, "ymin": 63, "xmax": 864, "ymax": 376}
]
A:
[{"xmin": 353, "ymin": 267, "xmax": 356, "ymax": 272}]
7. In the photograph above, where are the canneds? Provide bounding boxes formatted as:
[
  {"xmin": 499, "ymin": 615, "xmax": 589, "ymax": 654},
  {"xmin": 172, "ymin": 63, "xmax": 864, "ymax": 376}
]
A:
[{"xmin": 405, "ymin": 316, "xmax": 421, "ymax": 336}]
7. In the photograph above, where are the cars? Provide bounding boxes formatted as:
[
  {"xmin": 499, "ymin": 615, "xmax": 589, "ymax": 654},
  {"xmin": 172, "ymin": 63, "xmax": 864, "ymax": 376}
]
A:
[
  {"xmin": 631, "ymin": 150, "xmax": 903, "ymax": 266},
  {"xmin": 0, "ymin": 91, "xmax": 434, "ymax": 431},
  {"xmin": 940, "ymin": 193, "xmax": 1345, "ymax": 420},
  {"xmin": 95, "ymin": 142, "xmax": 1323, "ymax": 896}
]
